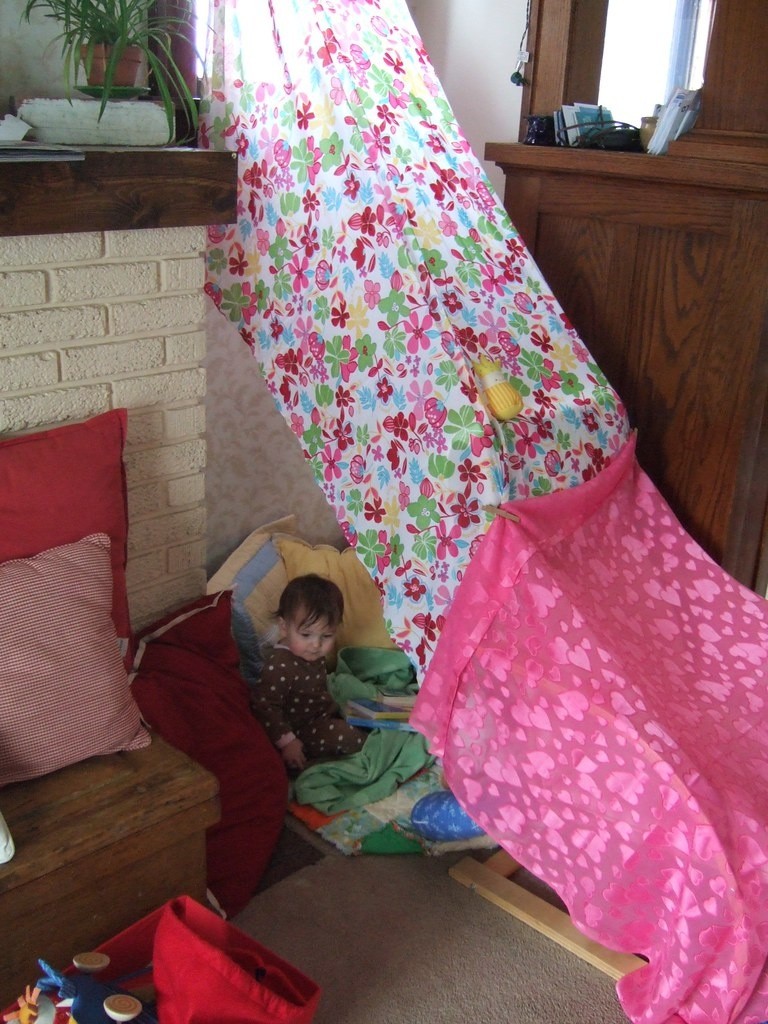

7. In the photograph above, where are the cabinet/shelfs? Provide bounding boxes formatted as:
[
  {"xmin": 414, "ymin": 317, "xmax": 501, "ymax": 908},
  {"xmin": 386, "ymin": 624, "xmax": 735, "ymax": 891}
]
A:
[
  {"xmin": 483, "ymin": 141, "xmax": 768, "ymax": 593},
  {"xmin": 0, "ymin": 734, "xmax": 221, "ymax": 1008}
]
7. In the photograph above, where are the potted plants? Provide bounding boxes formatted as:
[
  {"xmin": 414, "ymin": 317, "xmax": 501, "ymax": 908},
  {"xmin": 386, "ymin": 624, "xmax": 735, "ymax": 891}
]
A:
[{"xmin": 18, "ymin": 0, "xmax": 216, "ymax": 148}]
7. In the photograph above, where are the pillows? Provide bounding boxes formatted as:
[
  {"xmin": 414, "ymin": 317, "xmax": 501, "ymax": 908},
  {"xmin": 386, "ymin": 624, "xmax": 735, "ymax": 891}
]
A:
[
  {"xmin": 0, "ymin": 409, "xmax": 133, "ymax": 680},
  {"xmin": 273, "ymin": 532, "xmax": 403, "ymax": 651},
  {"xmin": 0, "ymin": 531, "xmax": 152, "ymax": 783},
  {"xmin": 127, "ymin": 588, "xmax": 287, "ymax": 920},
  {"xmin": 206, "ymin": 514, "xmax": 301, "ymax": 687}
]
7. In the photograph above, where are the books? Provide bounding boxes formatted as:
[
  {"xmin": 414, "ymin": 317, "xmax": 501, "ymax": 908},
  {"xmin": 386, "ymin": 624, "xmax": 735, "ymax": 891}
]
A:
[
  {"xmin": 0, "ymin": 140, "xmax": 87, "ymax": 162},
  {"xmin": 552, "ymin": 87, "xmax": 702, "ymax": 155},
  {"xmin": 346, "ymin": 687, "xmax": 417, "ymax": 732}
]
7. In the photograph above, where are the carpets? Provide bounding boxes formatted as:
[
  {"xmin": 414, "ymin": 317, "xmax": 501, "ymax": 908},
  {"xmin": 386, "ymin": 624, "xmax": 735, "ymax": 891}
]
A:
[{"xmin": 228, "ymin": 814, "xmax": 632, "ymax": 1024}]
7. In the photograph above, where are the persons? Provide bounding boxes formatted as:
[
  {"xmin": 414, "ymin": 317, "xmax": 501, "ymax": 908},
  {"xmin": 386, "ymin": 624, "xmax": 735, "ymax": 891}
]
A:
[{"xmin": 251, "ymin": 574, "xmax": 368, "ymax": 771}]
